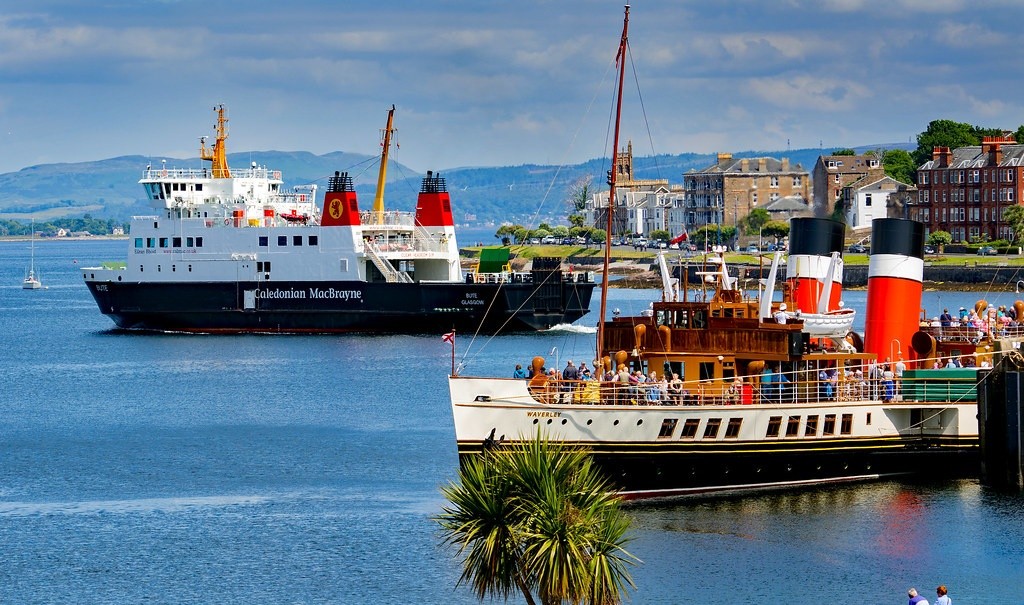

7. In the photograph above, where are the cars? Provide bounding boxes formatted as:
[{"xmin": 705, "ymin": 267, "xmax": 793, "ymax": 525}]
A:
[
  {"xmin": 529, "ymin": 233, "xmax": 600, "ymax": 245},
  {"xmin": 746, "ymin": 243, "xmax": 758, "ymax": 253},
  {"xmin": 924, "ymin": 248, "xmax": 934, "ymax": 254},
  {"xmin": 977, "ymin": 245, "xmax": 997, "ymax": 256},
  {"xmin": 611, "ymin": 234, "xmax": 680, "ymax": 251},
  {"xmin": 849, "ymin": 243, "xmax": 866, "ymax": 253}
]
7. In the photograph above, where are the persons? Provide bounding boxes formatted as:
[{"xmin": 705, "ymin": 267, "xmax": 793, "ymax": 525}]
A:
[
  {"xmin": 920, "ymin": 304, "xmax": 1020, "ymax": 345},
  {"xmin": 759, "ymin": 356, "xmax": 906, "ymax": 403},
  {"xmin": 513, "ymin": 360, "xmax": 685, "ymax": 406},
  {"xmin": 633, "ymin": 243, "xmax": 647, "ymax": 252},
  {"xmin": 933, "ymin": 357, "xmax": 991, "ymax": 369},
  {"xmin": 772, "ymin": 302, "xmax": 793, "ymax": 324}
]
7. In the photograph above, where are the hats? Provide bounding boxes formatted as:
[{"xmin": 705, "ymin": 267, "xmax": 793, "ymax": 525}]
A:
[
  {"xmin": 948, "ymin": 358, "xmax": 953, "ymax": 363},
  {"xmin": 959, "ymin": 307, "xmax": 965, "ymax": 310},
  {"xmin": 944, "ymin": 309, "xmax": 948, "ymax": 311},
  {"xmin": 780, "ymin": 303, "xmax": 786, "ymax": 310}
]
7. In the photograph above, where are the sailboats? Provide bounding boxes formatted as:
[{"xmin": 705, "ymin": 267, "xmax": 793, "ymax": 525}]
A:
[{"xmin": 22, "ymin": 218, "xmax": 43, "ymax": 289}]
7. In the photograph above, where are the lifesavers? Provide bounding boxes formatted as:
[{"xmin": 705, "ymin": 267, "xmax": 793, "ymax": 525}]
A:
[
  {"xmin": 274, "ymin": 172, "xmax": 280, "ymax": 179},
  {"xmin": 163, "ymin": 170, "xmax": 167, "ymax": 176},
  {"xmin": 224, "ymin": 219, "xmax": 229, "ymax": 225}
]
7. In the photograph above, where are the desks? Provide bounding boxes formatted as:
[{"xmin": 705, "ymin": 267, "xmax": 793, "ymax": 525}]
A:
[{"xmin": 466, "ymin": 272, "xmax": 533, "ymax": 283}]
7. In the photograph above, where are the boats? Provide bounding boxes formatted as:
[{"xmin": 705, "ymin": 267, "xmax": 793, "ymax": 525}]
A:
[
  {"xmin": 446, "ymin": 6, "xmax": 1024, "ymax": 507},
  {"xmin": 82, "ymin": 103, "xmax": 598, "ymax": 335}
]
